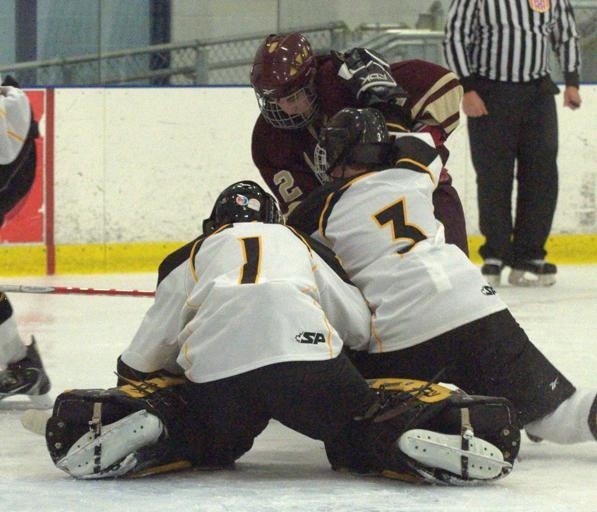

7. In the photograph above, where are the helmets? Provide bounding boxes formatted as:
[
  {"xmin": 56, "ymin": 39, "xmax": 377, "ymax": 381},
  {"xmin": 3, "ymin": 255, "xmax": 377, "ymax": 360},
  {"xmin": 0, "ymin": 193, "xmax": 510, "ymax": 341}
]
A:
[
  {"xmin": 202, "ymin": 180, "xmax": 283, "ymax": 235},
  {"xmin": 314, "ymin": 105, "xmax": 389, "ymax": 186},
  {"xmin": 249, "ymin": 31, "xmax": 319, "ymax": 129}
]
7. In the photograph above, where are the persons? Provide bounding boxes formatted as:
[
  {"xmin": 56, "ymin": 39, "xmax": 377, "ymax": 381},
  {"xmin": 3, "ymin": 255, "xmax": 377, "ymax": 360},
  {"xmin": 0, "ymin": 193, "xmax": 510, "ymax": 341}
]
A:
[
  {"xmin": 252, "ymin": 32, "xmax": 469, "ymax": 269},
  {"xmin": 285, "ymin": 49, "xmax": 597, "ymax": 443},
  {"xmin": 0, "ymin": 73, "xmax": 51, "ymax": 408},
  {"xmin": 443, "ymin": 0, "xmax": 584, "ymax": 286},
  {"xmin": 46, "ymin": 180, "xmax": 521, "ymax": 482}
]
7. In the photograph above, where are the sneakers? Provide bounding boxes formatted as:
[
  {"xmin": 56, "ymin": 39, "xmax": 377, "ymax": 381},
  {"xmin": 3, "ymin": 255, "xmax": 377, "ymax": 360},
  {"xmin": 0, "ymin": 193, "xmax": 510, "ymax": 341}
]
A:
[
  {"xmin": 507, "ymin": 255, "xmax": 557, "ymax": 275},
  {"xmin": 482, "ymin": 251, "xmax": 506, "ymax": 276},
  {"xmin": 0, "ymin": 336, "xmax": 52, "ymax": 402},
  {"xmin": 394, "ymin": 429, "xmax": 504, "ymax": 480},
  {"xmin": 66, "ymin": 409, "xmax": 164, "ymax": 479}
]
